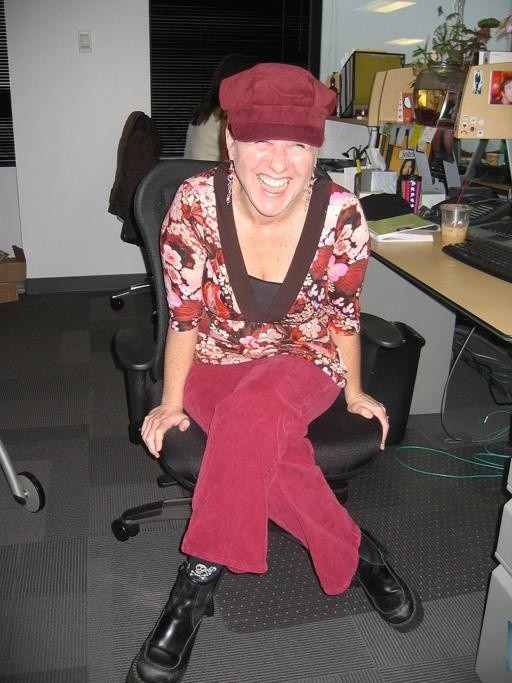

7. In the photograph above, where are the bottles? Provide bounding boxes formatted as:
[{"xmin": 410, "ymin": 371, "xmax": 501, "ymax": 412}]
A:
[{"xmin": 327, "ymin": 74, "xmax": 338, "ymax": 115}]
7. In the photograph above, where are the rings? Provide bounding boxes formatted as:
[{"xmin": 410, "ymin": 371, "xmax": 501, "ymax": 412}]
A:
[{"xmin": 386, "ymin": 416, "xmax": 389, "ymax": 419}]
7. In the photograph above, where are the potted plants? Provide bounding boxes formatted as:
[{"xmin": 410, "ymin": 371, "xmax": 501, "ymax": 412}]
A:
[{"xmin": 408, "ymin": 0, "xmax": 501, "ymax": 126}]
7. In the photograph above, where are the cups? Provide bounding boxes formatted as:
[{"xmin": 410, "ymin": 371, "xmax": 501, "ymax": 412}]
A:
[
  {"xmin": 438, "ymin": 203, "xmax": 475, "ymax": 251},
  {"xmin": 400, "ymin": 175, "xmax": 420, "ymax": 213}
]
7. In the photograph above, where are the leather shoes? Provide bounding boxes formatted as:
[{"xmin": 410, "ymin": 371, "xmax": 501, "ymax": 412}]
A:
[
  {"xmin": 356, "ymin": 532, "xmax": 423, "ymax": 636},
  {"xmin": 124, "ymin": 569, "xmax": 220, "ymax": 683}
]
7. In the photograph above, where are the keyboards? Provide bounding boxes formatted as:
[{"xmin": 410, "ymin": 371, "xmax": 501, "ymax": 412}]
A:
[{"xmin": 441, "ymin": 238, "xmax": 512, "ymax": 283}]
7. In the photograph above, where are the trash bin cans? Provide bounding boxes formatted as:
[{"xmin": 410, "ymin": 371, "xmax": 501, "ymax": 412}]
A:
[{"xmin": 362, "ymin": 321, "xmax": 427, "ymax": 448}]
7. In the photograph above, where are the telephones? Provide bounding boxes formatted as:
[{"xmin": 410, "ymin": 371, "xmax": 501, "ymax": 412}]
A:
[{"xmin": 425, "ymin": 194, "xmax": 512, "ymax": 227}]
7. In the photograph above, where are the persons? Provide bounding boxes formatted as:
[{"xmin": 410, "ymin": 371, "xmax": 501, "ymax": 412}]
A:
[
  {"xmin": 123, "ymin": 63, "xmax": 423, "ymax": 683},
  {"xmin": 494, "ymin": 76, "xmax": 512, "ymax": 105},
  {"xmin": 400, "ymin": 160, "xmax": 416, "ymax": 176},
  {"xmin": 184, "ymin": 54, "xmax": 255, "ymax": 163},
  {"xmin": 474, "ymin": 71, "xmax": 481, "ymax": 93}
]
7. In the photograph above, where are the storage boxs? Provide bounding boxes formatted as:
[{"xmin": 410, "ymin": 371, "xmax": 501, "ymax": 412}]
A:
[
  {"xmin": 339, "ymin": 51, "xmax": 405, "ymax": 118},
  {"xmin": 0, "ymin": 245, "xmax": 26, "ymax": 303}
]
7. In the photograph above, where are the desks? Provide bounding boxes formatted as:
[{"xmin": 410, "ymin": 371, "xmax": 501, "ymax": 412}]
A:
[{"xmin": 370, "ymin": 232, "xmax": 512, "ymax": 343}]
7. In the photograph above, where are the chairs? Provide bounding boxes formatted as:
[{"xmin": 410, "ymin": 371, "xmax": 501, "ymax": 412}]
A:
[
  {"xmin": 112, "ymin": 160, "xmax": 404, "ymax": 616},
  {"xmin": 109, "ymin": 111, "xmax": 160, "ymax": 311}
]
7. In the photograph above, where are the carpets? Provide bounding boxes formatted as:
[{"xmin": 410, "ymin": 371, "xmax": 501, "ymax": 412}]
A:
[{"xmin": 213, "ymin": 426, "xmax": 512, "ymax": 634}]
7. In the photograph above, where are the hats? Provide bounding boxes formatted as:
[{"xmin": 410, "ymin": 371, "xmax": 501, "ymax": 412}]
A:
[{"xmin": 218, "ymin": 60, "xmax": 338, "ymax": 146}]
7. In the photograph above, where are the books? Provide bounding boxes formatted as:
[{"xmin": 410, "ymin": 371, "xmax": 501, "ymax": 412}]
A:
[{"xmin": 366, "ymin": 213, "xmax": 436, "ymax": 243}]
